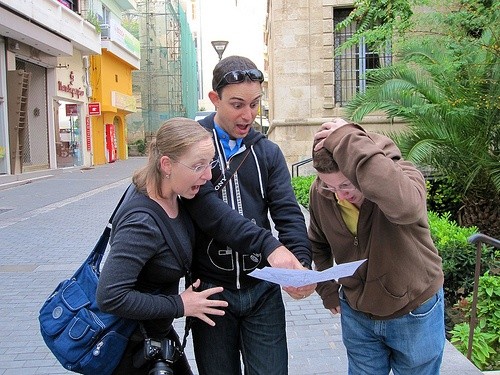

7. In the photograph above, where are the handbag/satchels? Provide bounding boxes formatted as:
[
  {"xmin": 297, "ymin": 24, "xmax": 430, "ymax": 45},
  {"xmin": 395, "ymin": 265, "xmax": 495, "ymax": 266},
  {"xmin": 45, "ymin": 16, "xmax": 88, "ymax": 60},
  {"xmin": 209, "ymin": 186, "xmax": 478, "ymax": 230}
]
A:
[{"xmin": 39, "ymin": 182, "xmax": 137, "ymax": 375}]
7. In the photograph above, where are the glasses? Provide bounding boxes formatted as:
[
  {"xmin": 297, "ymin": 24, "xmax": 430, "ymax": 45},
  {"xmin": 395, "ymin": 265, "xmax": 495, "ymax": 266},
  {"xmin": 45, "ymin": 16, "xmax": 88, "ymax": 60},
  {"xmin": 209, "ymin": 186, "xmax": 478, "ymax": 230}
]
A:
[
  {"xmin": 214, "ymin": 70, "xmax": 264, "ymax": 90},
  {"xmin": 173, "ymin": 159, "xmax": 219, "ymax": 173},
  {"xmin": 321, "ymin": 180, "xmax": 357, "ymax": 191}
]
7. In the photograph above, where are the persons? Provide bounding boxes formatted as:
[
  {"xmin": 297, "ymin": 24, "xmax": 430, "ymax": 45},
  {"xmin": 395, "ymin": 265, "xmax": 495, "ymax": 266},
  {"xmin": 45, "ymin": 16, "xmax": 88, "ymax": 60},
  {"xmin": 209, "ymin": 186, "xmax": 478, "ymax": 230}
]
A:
[
  {"xmin": 307, "ymin": 119, "xmax": 445, "ymax": 375},
  {"xmin": 184, "ymin": 55, "xmax": 307, "ymax": 375},
  {"xmin": 98, "ymin": 118, "xmax": 318, "ymax": 375}
]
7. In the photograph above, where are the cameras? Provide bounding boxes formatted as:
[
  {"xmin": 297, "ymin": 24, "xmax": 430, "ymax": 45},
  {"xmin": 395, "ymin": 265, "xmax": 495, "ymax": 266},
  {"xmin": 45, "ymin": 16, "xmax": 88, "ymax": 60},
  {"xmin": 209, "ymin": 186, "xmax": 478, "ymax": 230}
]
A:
[{"xmin": 132, "ymin": 337, "xmax": 184, "ymax": 375}]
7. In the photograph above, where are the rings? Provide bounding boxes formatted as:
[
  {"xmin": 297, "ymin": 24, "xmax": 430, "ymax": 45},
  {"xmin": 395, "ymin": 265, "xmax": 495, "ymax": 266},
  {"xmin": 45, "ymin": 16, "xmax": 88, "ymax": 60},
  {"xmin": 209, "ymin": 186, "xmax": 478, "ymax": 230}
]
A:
[{"xmin": 303, "ymin": 295, "xmax": 306, "ymax": 299}]
